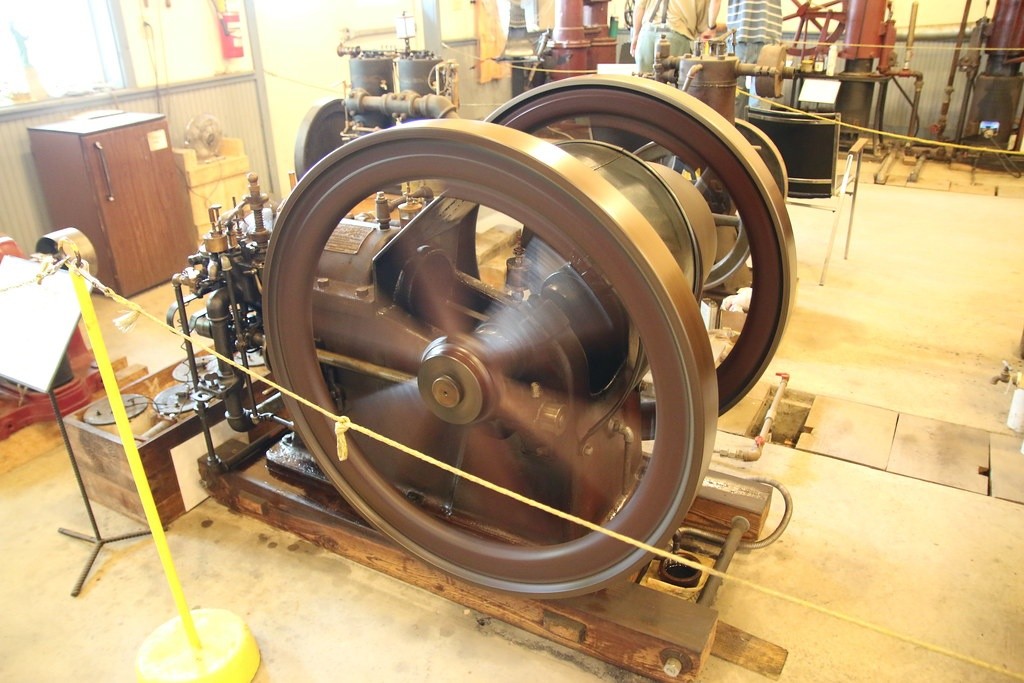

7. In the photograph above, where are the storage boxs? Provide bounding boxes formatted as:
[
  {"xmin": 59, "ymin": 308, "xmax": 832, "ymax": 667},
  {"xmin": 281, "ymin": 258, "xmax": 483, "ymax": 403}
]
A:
[{"xmin": 62, "ymin": 341, "xmax": 292, "ymax": 533}]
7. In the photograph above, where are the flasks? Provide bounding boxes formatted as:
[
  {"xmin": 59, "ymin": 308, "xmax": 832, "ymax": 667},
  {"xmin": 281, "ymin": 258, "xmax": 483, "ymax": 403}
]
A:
[{"xmin": 610, "ymin": 17, "xmax": 619, "ymax": 38}]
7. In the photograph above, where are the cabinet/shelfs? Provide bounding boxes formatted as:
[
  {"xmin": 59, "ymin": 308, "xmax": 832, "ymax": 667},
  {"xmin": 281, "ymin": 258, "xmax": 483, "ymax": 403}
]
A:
[{"xmin": 27, "ymin": 110, "xmax": 200, "ymax": 298}]
